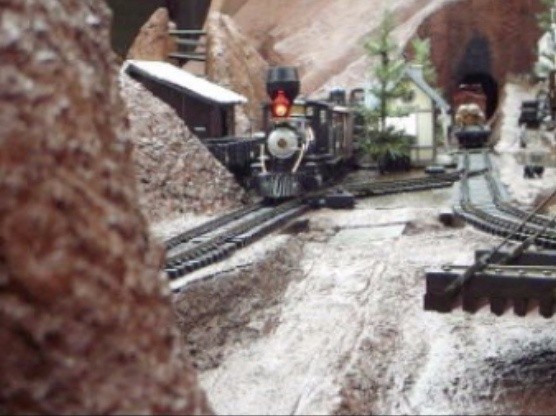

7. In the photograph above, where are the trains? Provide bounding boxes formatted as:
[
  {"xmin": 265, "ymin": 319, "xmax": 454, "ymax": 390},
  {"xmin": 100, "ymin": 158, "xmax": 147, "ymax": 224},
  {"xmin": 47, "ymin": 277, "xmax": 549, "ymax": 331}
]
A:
[
  {"xmin": 455, "ymin": 83, "xmax": 491, "ymax": 149},
  {"xmin": 258, "ymin": 65, "xmax": 365, "ymax": 198}
]
